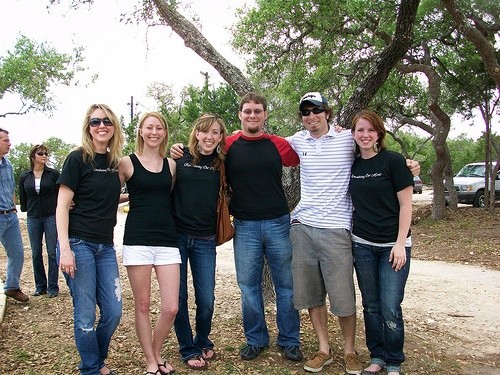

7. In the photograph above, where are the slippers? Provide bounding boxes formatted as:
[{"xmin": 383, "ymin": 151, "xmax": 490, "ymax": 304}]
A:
[
  {"xmin": 201, "ymin": 347, "xmax": 217, "ymax": 360},
  {"xmin": 184, "ymin": 355, "xmax": 208, "ymax": 370},
  {"xmin": 145, "ymin": 370, "xmax": 162, "ymax": 375},
  {"xmin": 158, "ymin": 361, "xmax": 176, "ymax": 374}
]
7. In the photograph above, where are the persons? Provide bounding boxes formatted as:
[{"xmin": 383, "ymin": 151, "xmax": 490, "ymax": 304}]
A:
[
  {"xmin": 285, "ymin": 91, "xmax": 420, "ymax": 375},
  {"xmin": 55, "ymin": 104, "xmax": 123, "ymax": 375},
  {"xmin": 222, "ymin": 92, "xmax": 347, "ymax": 361},
  {"xmin": 69, "ymin": 113, "xmax": 183, "ymax": 375},
  {"xmin": 168, "ymin": 113, "xmax": 227, "ymax": 367},
  {"xmin": 0, "ymin": 128, "xmax": 30, "ymax": 303},
  {"xmin": 347, "ymin": 110, "xmax": 414, "ymax": 375},
  {"xmin": 19, "ymin": 145, "xmax": 61, "ymax": 298}
]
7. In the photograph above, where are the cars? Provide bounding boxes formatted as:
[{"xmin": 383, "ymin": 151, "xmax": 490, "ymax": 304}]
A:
[{"xmin": 412, "ymin": 175, "xmax": 422, "ymax": 194}]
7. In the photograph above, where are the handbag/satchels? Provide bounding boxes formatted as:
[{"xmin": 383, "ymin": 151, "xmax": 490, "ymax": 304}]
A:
[{"xmin": 215, "ymin": 158, "xmax": 234, "ymax": 245}]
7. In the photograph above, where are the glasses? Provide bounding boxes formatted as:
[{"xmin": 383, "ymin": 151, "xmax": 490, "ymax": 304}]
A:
[
  {"xmin": 241, "ymin": 109, "xmax": 264, "ymax": 114},
  {"xmin": 37, "ymin": 151, "xmax": 48, "ymax": 155},
  {"xmin": 301, "ymin": 108, "xmax": 325, "ymax": 116},
  {"xmin": 89, "ymin": 116, "xmax": 114, "ymax": 126}
]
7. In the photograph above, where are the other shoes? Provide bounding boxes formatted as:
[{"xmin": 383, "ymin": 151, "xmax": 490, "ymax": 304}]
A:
[
  {"xmin": 277, "ymin": 339, "xmax": 302, "ymax": 360},
  {"xmin": 240, "ymin": 343, "xmax": 269, "ymax": 359},
  {"xmin": 361, "ymin": 364, "xmax": 384, "ymax": 374},
  {"xmin": 303, "ymin": 348, "xmax": 334, "ymax": 372},
  {"xmin": 4, "ymin": 288, "xmax": 30, "ymax": 302},
  {"xmin": 33, "ymin": 288, "xmax": 47, "ymax": 295},
  {"xmin": 49, "ymin": 290, "xmax": 58, "ymax": 297},
  {"xmin": 102, "ymin": 370, "xmax": 118, "ymax": 375},
  {"xmin": 343, "ymin": 350, "xmax": 364, "ymax": 374}
]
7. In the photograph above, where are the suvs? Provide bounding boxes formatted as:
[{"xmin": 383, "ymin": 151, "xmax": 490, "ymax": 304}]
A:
[{"xmin": 443, "ymin": 161, "xmax": 500, "ymax": 209}]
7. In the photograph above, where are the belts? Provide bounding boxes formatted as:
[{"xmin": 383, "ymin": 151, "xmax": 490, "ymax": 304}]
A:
[{"xmin": 1, "ymin": 208, "xmax": 13, "ymax": 214}]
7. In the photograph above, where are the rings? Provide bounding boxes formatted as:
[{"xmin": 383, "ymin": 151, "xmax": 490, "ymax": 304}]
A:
[
  {"xmin": 61, "ymin": 268, "xmax": 64, "ymax": 270},
  {"xmin": 71, "ymin": 267, "xmax": 74, "ymax": 269}
]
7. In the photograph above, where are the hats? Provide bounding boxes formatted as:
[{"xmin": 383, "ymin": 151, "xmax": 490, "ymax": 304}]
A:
[{"xmin": 299, "ymin": 91, "xmax": 328, "ymax": 109}]
